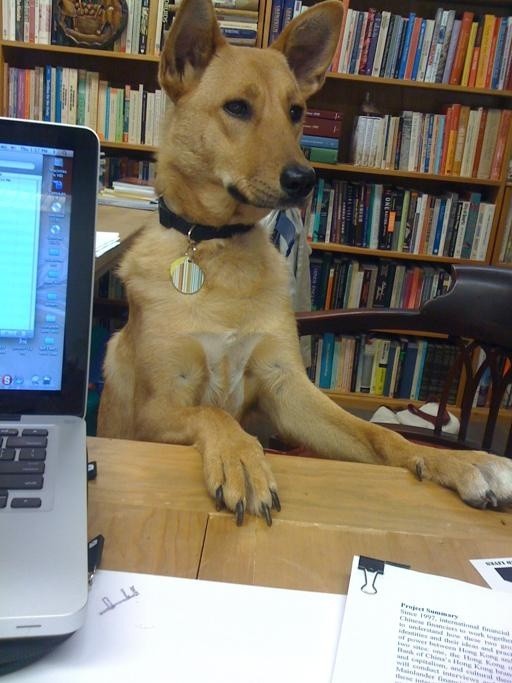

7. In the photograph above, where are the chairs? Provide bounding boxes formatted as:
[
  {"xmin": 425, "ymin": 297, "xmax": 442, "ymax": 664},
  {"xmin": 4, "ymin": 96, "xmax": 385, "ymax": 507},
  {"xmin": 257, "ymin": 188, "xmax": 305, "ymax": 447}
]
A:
[{"xmin": 264, "ymin": 264, "xmax": 512, "ymax": 457}]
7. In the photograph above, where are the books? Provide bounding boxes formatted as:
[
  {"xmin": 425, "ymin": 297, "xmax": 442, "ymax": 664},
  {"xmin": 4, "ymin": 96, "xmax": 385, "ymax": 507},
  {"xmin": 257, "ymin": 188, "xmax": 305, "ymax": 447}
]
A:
[
  {"xmin": 269, "ymin": 1, "xmax": 511, "ymax": 91},
  {"xmin": 300, "ymin": 178, "xmax": 512, "ymax": 407},
  {"xmin": 301, "ymin": 103, "xmax": 511, "ymax": 179},
  {"xmin": 97, "ymin": 152, "xmax": 158, "ymax": 210},
  {"xmin": 96, "ymin": 232, "xmax": 119, "ymax": 257},
  {"xmin": 1, "ymin": 1, "xmax": 258, "ymax": 56},
  {"xmin": 1, "ymin": 61, "xmax": 166, "ymax": 147}
]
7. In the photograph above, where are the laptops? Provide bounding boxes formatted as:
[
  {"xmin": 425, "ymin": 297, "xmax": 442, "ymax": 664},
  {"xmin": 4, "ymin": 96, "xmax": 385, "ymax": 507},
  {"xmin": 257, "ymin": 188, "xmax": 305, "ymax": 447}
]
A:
[{"xmin": 0, "ymin": 116, "xmax": 101, "ymax": 667}]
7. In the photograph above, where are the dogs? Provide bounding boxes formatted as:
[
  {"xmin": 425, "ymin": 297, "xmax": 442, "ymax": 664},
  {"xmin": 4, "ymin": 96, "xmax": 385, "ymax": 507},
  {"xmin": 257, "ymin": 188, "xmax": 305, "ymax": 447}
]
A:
[{"xmin": 96, "ymin": 0, "xmax": 512, "ymax": 530}]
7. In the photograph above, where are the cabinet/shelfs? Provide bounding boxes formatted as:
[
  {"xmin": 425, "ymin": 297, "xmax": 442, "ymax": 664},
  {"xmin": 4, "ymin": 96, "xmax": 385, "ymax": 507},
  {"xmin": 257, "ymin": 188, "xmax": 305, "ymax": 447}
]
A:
[{"xmin": 0, "ymin": 0, "xmax": 512, "ymax": 424}]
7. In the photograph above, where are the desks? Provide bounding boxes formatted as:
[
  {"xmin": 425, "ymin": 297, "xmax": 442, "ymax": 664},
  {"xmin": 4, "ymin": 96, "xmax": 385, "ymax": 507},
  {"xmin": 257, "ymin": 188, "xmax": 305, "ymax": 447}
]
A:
[
  {"xmin": 87, "ymin": 436, "xmax": 512, "ymax": 594},
  {"xmin": 94, "ymin": 203, "xmax": 155, "ymax": 283}
]
